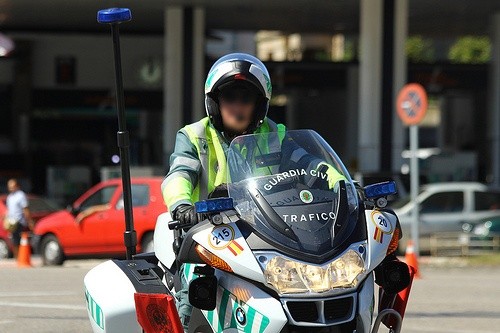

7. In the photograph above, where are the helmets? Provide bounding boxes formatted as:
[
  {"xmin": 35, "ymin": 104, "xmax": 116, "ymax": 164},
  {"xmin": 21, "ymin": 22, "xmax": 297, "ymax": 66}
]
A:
[{"xmin": 205, "ymin": 52, "xmax": 272, "ymax": 137}]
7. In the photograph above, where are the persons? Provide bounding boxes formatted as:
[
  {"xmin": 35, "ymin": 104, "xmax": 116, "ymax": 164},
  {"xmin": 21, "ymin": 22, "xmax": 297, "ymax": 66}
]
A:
[
  {"xmin": 162, "ymin": 52, "xmax": 376, "ymax": 232},
  {"xmin": 6, "ymin": 179, "xmax": 35, "ymax": 260}
]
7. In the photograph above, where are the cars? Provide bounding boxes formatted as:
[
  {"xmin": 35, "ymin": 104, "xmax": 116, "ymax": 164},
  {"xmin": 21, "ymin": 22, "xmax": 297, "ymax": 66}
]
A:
[
  {"xmin": 0, "ymin": 194, "xmax": 58, "ymax": 259},
  {"xmin": 31, "ymin": 176, "xmax": 166, "ymax": 265},
  {"xmin": 384, "ymin": 181, "xmax": 498, "ymax": 252}
]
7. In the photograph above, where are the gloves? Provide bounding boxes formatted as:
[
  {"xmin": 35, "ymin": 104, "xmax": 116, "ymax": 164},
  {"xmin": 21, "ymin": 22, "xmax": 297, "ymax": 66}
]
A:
[{"xmin": 173, "ymin": 205, "xmax": 219, "ymax": 232}]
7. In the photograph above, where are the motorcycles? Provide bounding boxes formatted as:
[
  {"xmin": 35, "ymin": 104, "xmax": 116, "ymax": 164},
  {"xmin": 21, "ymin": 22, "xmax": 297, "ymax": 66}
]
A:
[{"xmin": 81, "ymin": 5, "xmax": 404, "ymax": 332}]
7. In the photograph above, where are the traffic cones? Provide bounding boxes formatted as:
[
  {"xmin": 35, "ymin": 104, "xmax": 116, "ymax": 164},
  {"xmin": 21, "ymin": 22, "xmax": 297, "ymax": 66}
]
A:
[
  {"xmin": 404, "ymin": 240, "xmax": 421, "ymax": 278},
  {"xmin": 18, "ymin": 232, "xmax": 34, "ymax": 268}
]
